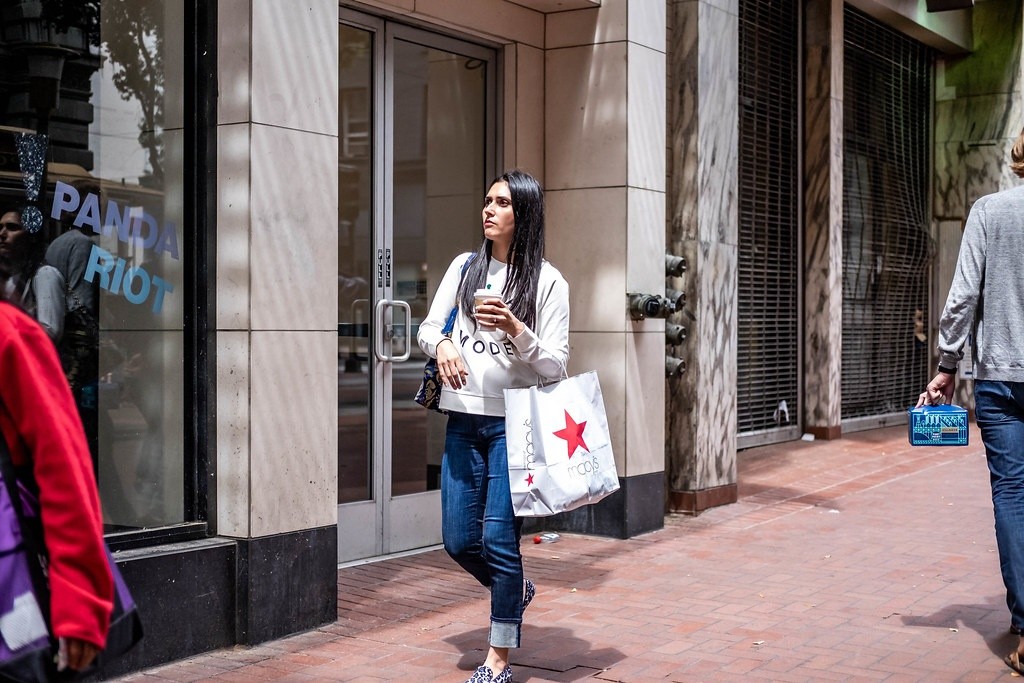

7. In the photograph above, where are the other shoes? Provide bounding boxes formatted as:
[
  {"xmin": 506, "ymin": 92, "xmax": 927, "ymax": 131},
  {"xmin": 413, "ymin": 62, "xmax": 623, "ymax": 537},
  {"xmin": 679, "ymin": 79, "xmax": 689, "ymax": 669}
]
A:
[
  {"xmin": 464, "ymin": 664, "xmax": 513, "ymax": 683},
  {"xmin": 522, "ymin": 578, "xmax": 535, "ymax": 615}
]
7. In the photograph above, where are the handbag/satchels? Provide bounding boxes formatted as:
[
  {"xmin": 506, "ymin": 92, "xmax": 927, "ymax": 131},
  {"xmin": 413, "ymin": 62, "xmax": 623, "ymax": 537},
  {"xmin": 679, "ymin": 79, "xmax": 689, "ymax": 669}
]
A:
[
  {"xmin": 502, "ymin": 364, "xmax": 619, "ymax": 517},
  {"xmin": 414, "ymin": 304, "xmax": 459, "ymax": 413},
  {"xmin": 0, "ymin": 472, "xmax": 146, "ymax": 683}
]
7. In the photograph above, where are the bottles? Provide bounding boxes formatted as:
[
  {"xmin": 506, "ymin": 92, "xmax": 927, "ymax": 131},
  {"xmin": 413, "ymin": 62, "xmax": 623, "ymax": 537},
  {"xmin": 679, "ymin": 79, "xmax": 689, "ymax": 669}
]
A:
[{"xmin": 533, "ymin": 533, "xmax": 560, "ymax": 544}]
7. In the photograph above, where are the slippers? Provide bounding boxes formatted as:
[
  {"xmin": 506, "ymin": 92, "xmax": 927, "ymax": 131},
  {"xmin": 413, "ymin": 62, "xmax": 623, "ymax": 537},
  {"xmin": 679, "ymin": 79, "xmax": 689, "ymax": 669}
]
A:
[{"xmin": 1004, "ymin": 649, "xmax": 1024, "ymax": 677}]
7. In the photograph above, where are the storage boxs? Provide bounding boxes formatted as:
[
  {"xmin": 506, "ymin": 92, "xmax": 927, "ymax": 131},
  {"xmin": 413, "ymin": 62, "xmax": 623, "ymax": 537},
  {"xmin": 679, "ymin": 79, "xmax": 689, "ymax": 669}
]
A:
[{"xmin": 907, "ymin": 404, "xmax": 970, "ymax": 446}]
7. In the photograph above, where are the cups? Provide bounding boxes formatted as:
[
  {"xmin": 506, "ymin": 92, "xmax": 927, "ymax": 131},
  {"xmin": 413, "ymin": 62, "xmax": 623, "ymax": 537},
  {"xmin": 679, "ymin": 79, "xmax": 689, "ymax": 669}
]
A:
[{"xmin": 473, "ymin": 289, "xmax": 502, "ymax": 331}]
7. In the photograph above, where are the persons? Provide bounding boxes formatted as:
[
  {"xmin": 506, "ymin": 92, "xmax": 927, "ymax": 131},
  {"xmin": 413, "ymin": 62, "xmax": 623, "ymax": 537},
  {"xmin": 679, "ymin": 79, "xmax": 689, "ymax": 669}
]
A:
[
  {"xmin": 1, "ymin": 179, "xmax": 101, "ymax": 344},
  {"xmin": 916, "ymin": 131, "xmax": 1023, "ymax": 669},
  {"xmin": 417, "ymin": 168, "xmax": 569, "ymax": 683},
  {"xmin": 0, "ymin": 302, "xmax": 113, "ymax": 683}
]
